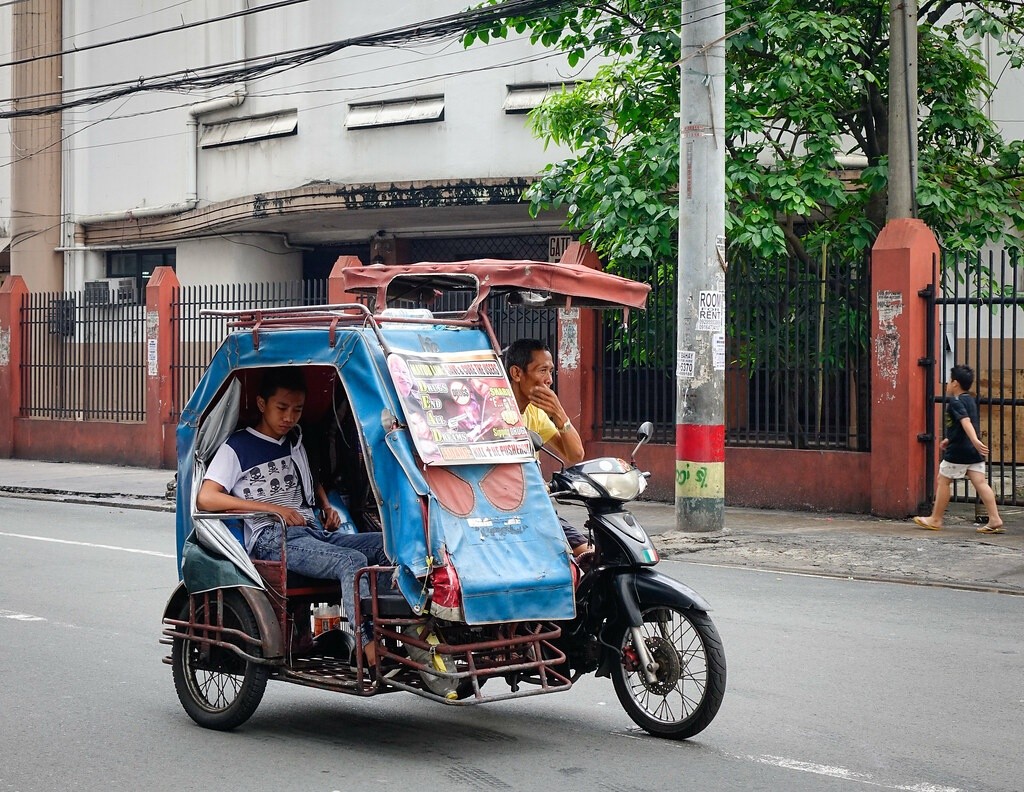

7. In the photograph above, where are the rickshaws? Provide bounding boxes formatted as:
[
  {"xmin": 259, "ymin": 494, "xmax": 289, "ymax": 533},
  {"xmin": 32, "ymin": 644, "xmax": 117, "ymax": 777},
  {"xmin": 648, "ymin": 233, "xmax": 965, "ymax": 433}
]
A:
[{"xmin": 160, "ymin": 258, "xmax": 728, "ymax": 741}]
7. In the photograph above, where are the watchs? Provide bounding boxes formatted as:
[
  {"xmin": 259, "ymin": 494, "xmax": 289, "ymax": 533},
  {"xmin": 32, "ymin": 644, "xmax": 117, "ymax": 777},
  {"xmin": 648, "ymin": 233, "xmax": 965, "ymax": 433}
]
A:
[{"xmin": 557, "ymin": 419, "xmax": 571, "ymax": 432}]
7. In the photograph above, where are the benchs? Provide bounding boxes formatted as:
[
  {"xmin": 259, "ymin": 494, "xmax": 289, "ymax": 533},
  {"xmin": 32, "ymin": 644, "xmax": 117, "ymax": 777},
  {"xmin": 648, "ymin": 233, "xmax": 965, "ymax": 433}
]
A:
[{"xmin": 220, "ymin": 489, "xmax": 376, "ymax": 594}]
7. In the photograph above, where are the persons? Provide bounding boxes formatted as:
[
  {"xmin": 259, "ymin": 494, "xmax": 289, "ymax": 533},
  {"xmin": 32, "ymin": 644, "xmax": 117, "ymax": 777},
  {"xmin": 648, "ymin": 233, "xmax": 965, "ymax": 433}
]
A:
[
  {"xmin": 196, "ymin": 367, "xmax": 399, "ymax": 679},
  {"xmin": 913, "ymin": 363, "xmax": 1007, "ymax": 533},
  {"xmin": 504, "ymin": 337, "xmax": 595, "ymax": 559}
]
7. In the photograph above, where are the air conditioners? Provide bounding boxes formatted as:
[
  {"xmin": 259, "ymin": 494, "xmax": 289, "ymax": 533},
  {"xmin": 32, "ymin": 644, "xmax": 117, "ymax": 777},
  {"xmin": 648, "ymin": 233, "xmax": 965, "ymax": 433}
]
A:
[{"xmin": 83, "ymin": 277, "xmax": 137, "ymax": 306}]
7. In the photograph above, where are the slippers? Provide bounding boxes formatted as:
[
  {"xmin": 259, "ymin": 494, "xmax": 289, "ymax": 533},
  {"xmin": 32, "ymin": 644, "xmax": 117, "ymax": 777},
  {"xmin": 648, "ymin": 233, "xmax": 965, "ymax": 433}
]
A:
[
  {"xmin": 976, "ymin": 526, "xmax": 1006, "ymax": 534},
  {"xmin": 914, "ymin": 517, "xmax": 941, "ymax": 531}
]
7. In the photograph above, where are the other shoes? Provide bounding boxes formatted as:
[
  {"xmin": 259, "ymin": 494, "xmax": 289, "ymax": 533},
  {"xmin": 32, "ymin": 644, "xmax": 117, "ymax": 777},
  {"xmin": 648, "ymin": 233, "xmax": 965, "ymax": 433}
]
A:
[{"xmin": 369, "ymin": 652, "xmax": 412, "ymax": 685}]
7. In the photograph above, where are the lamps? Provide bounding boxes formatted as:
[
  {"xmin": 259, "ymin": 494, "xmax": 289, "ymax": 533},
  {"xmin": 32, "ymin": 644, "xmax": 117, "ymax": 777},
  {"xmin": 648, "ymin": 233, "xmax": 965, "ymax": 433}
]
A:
[{"xmin": 371, "ymin": 251, "xmax": 384, "ymax": 263}]
